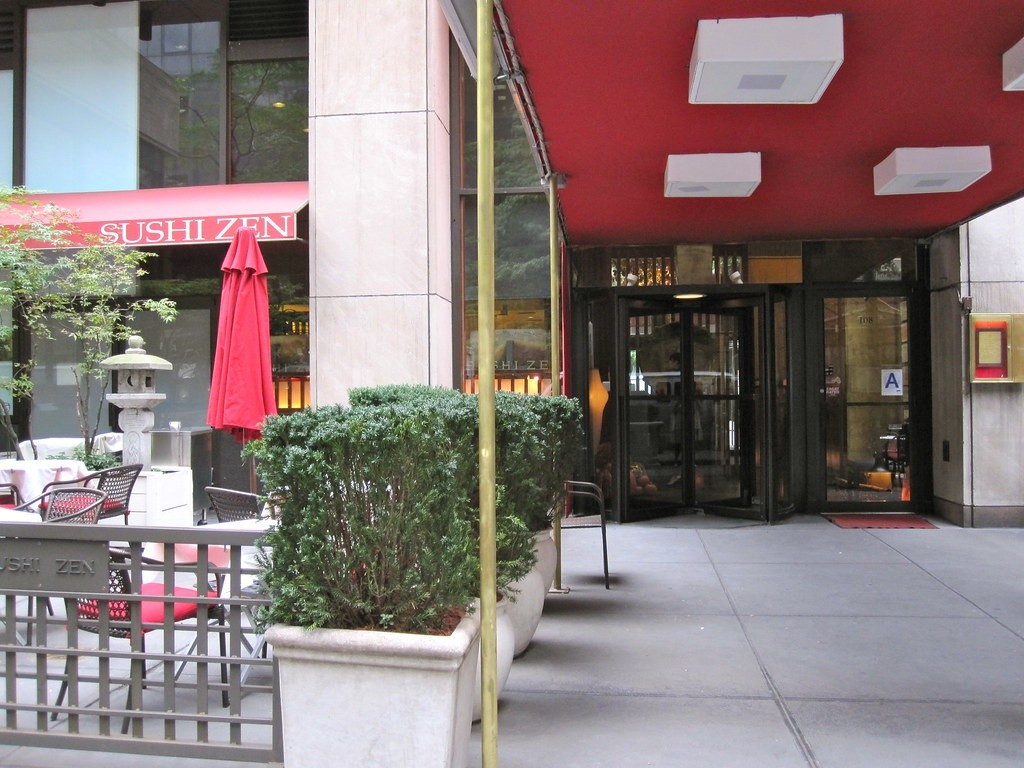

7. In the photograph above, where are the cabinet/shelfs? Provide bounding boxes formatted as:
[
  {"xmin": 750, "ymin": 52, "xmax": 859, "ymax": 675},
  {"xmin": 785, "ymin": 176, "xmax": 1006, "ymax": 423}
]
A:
[{"xmin": 150, "ymin": 427, "xmax": 212, "ymax": 511}]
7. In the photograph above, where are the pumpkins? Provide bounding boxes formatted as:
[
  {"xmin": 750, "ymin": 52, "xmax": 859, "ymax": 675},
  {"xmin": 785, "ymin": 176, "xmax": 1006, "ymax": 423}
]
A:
[{"xmin": 635, "ymin": 475, "xmax": 659, "ymax": 497}]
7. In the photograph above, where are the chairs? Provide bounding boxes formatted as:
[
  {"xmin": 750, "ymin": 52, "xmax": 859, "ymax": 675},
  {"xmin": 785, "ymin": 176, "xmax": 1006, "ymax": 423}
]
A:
[
  {"xmin": 39, "ymin": 464, "xmax": 143, "ymax": 525},
  {"xmin": 11, "ymin": 486, "xmax": 107, "ymax": 617},
  {"xmin": 205, "ymin": 486, "xmax": 275, "ymax": 662},
  {"xmin": 48, "ymin": 546, "xmax": 227, "ymax": 734}
]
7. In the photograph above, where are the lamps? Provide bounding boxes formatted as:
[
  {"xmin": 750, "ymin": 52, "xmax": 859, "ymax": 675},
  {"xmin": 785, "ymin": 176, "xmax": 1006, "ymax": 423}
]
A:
[
  {"xmin": 626, "ymin": 274, "xmax": 638, "ymax": 286},
  {"xmin": 730, "ymin": 271, "xmax": 744, "ymax": 284}
]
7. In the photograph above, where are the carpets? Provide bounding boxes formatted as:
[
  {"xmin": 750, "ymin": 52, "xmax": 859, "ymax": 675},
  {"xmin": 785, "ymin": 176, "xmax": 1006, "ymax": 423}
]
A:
[{"xmin": 820, "ymin": 513, "xmax": 938, "ymax": 528}]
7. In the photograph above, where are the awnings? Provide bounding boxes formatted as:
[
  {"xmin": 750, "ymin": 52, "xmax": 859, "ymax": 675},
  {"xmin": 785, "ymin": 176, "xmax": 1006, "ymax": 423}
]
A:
[{"xmin": 0, "ymin": 181, "xmax": 309, "ymax": 251}]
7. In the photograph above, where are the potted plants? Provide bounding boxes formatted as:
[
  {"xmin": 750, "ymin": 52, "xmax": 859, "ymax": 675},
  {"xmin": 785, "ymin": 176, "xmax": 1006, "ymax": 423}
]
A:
[{"xmin": 238, "ymin": 382, "xmax": 586, "ymax": 768}]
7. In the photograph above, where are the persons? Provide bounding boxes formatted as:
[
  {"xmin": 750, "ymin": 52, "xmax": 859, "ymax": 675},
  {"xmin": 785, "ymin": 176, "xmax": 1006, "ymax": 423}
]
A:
[{"xmin": 665, "ymin": 353, "xmax": 704, "ymax": 486}]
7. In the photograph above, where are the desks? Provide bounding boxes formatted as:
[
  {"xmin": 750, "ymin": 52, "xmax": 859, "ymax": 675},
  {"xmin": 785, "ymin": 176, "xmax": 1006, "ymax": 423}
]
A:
[
  {"xmin": 0, "ymin": 457, "xmax": 95, "ymax": 508},
  {"xmin": 20, "ymin": 432, "xmax": 122, "ymax": 460},
  {"xmin": 197, "ymin": 517, "xmax": 282, "ymax": 532}
]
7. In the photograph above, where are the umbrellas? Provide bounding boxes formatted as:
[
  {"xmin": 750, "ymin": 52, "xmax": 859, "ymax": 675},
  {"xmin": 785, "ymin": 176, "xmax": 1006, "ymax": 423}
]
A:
[{"xmin": 206, "ymin": 227, "xmax": 278, "ymax": 492}]
